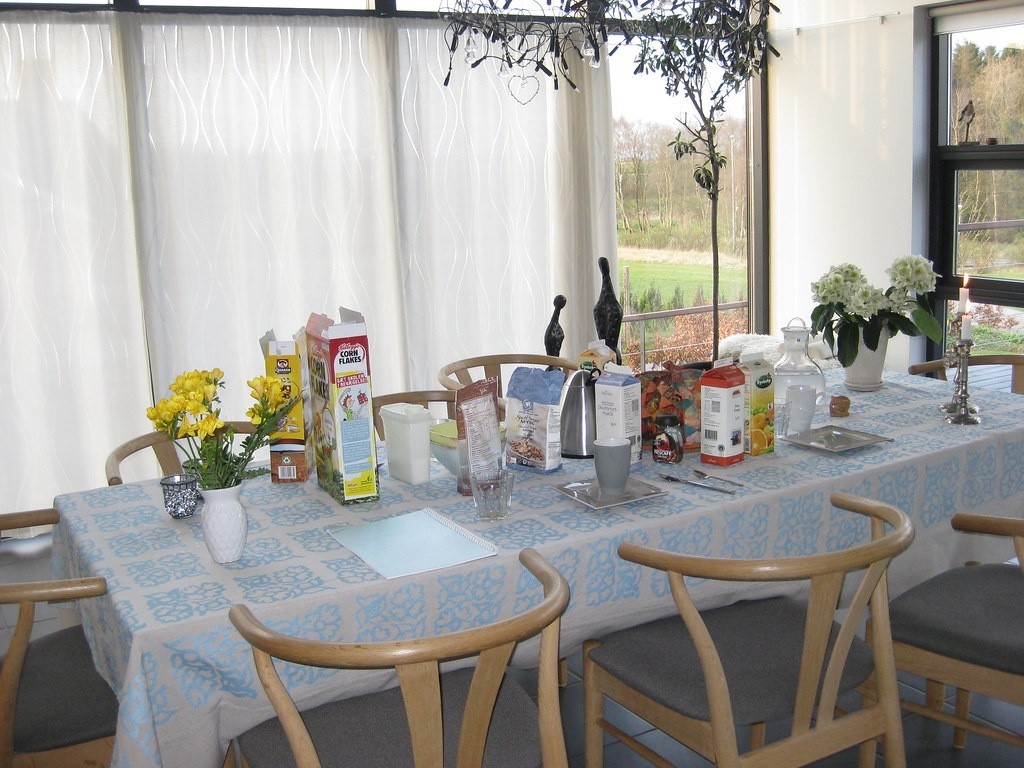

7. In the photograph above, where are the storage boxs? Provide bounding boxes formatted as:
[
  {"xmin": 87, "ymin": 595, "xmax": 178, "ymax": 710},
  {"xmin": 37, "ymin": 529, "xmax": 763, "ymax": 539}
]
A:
[
  {"xmin": 595, "ymin": 361, "xmax": 642, "ymax": 464},
  {"xmin": 306, "ymin": 306, "xmax": 380, "ymax": 505},
  {"xmin": 259, "ymin": 325, "xmax": 315, "ymax": 483},
  {"xmin": 700, "ymin": 357, "xmax": 746, "ymax": 467},
  {"xmin": 737, "ymin": 352, "xmax": 776, "ymax": 456}
]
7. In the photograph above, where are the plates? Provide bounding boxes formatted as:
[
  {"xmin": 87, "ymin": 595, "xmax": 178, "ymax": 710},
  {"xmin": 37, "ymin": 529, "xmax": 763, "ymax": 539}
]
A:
[
  {"xmin": 550, "ymin": 475, "xmax": 668, "ymax": 511},
  {"xmin": 780, "ymin": 424, "xmax": 894, "ymax": 453}
]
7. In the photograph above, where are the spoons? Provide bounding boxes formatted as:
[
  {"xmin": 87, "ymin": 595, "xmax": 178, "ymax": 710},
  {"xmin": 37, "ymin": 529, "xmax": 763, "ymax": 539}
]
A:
[{"xmin": 694, "ymin": 469, "xmax": 744, "ymax": 487}]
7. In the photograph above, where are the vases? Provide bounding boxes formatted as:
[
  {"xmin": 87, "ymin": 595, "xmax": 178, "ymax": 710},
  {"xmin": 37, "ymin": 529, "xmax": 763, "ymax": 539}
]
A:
[
  {"xmin": 843, "ymin": 327, "xmax": 888, "ymax": 391},
  {"xmin": 196, "ymin": 480, "xmax": 249, "ymax": 563}
]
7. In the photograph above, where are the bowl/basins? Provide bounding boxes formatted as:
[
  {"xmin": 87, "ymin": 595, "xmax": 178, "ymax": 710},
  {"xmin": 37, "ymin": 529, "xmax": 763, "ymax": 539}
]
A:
[
  {"xmin": 429, "ymin": 420, "xmax": 507, "ymax": 449},
  {"xmin": 430, "ymin": 438, "xmax": 509, "ymax": 476}
]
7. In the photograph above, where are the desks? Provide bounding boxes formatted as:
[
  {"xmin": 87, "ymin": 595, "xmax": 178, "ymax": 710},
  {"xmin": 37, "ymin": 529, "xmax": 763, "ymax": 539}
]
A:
[{"xmin": 39, "ymin": 359, "xmax": 1024, "ymax": 768}]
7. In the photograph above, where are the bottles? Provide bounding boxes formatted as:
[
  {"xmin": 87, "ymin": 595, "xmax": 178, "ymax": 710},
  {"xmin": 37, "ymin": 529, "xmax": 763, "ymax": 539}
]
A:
[
  {"xmin": 652, "ymin": 412, "xmax": 683, "ymax": 464},
  {"xmin": 773, "ymin": 316, "xmax": 826, "ymax": 413}
]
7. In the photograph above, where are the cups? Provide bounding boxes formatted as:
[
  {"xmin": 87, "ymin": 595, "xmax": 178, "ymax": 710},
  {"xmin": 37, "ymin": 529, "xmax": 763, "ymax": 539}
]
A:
[
  {"xmin": 593, "ymin": 436, "xmax": 630, "ymax": 497},
  {"xmin": 774, "ymin": 397, "xmax": 792, "ymax": 438},
  {"xmin": 160, "ymin": 474, "xmax": 198, "ymax": 519},
  {"xmin": 786, "ymin": 384, "xmax": 827, "ymax": 431},
  {"xmin": 182, "ymin": 458, "xmax": 209, "ymax": 497},
  {"xmin": 468, "ymin": 469, "xmax": 515, "ymax": 520}
]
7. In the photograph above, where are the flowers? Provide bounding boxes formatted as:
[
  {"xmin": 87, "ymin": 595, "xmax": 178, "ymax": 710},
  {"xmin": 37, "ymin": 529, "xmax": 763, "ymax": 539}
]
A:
[
  {"xmin": 810, "ymin": 254, "xmax": 942, "ymax": 368},
  {"xmin": 146, "ymin": 366, "xmax": 309, "ymax": 479}
]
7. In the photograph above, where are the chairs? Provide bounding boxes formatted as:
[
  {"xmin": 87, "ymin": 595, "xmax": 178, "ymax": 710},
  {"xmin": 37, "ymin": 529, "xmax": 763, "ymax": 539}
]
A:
[
  {"xmin": 583, "ymin": 490, "xmax": 913, "ymax": 768},
  {"xmin": 372, "ymin": 390, "xmax": 457, "ymax": 440},
  {"xmin": 104, "ymin": 420, "xmax": 260, "ymax": 485},
  {"xmin": 438, "ymin": 354, "xmax": 580, "ymax": 422},
  {"xmin": 225, "ymin": 547, "xmax": 570, "ymax": 768},
  {"xmin": 0, "ymin": 509, "xmax": 249, "ymax": 768},
  {"xmin": 908, "ymin": 355, "xmax": 1024, "ymax": 395},
  {"xmin": 861, "ymin": 513, "xmax": 1024, "ymax": 768}
]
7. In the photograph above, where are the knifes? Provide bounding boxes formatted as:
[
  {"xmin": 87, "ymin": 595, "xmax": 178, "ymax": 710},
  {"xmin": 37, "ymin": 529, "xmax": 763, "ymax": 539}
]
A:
[{"xmin": 657, "ymin": 473, "xmax": 735, "ymax": 495}]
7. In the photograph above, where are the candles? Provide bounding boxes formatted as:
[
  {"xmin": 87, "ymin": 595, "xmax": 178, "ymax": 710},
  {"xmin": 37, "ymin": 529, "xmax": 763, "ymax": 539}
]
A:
[
  {"xmin": 961, "ymin": 298, "xmax": 972, "ymax": 339},
  {"xmin": 959, "ymin": 272, "xmax": 971, "ymax": 313}
]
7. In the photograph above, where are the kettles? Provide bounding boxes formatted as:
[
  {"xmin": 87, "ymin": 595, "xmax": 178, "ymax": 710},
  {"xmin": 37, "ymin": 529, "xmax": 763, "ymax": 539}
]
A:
[{"xmin": 560, "ymin": 367, "xmax": 597, "ymax": 459}]
